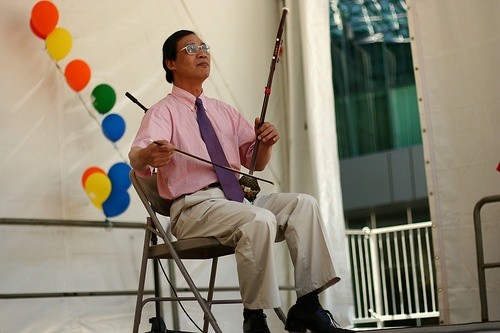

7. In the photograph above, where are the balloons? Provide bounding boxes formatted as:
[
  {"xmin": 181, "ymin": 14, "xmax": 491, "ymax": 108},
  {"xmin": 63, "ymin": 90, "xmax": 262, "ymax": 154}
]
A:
[
  {"xmin": 63, "ymin": 59, "xmax": 92, "ymax": 93},
  {"xmin": 91, "ymin": 84, "xmax": 117, "ymax": 115},
  {"xmin": 81, "ymin": 166, "xmax": 112, "ymax": 209},
  {"xmin": 43, "ymin": 27, "xmax": 73, "ymax": 62},
  {"xmin": 101, "ymin": 113, "xmax": 126, "ymax": 144},
  {"xmin": 102, "ymin": 191, "xmax": 131, "ymax": 217},
  {"xmin": 107, "ymin": 162, "xmax": 136, "ymax": 192},
  {"xmin": 28, "ymin": 0, "xmax": 59, "ymax": 39}
]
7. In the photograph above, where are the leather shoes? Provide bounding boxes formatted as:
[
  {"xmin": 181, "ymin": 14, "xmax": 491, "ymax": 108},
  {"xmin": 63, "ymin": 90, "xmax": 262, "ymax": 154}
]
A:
[
  {"xmin": 284, "ymin": 303, "xmax": 355, "ymax": 333},
  {"xmin": 243, "ymin": 316, "xmax": 272, "ymax": 333}
]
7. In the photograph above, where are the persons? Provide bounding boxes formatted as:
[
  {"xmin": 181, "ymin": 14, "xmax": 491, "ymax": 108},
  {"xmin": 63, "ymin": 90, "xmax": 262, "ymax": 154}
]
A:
[{"xmin": 128, "ymin": 29, "xmax": 355, "ymax": 333}]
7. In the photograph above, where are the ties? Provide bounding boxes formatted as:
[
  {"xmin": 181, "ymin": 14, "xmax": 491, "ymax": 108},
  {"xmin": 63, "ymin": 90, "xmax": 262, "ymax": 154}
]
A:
[{"xmin": 196, "ymin": 97, "xmax": 245, "ymax": 203}]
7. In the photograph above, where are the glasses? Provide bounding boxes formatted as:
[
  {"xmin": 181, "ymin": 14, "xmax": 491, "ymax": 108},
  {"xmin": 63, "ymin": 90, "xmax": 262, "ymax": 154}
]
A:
[{"xmin": 176, "ymin": 43, "xmax": 211, "ymax": 55}]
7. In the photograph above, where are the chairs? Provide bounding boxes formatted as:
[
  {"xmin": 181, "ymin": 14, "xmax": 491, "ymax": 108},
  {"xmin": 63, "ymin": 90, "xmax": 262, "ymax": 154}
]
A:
[{"xmin": 130, "ymin": 169, "xmax": 287, "ymax": 333}]
